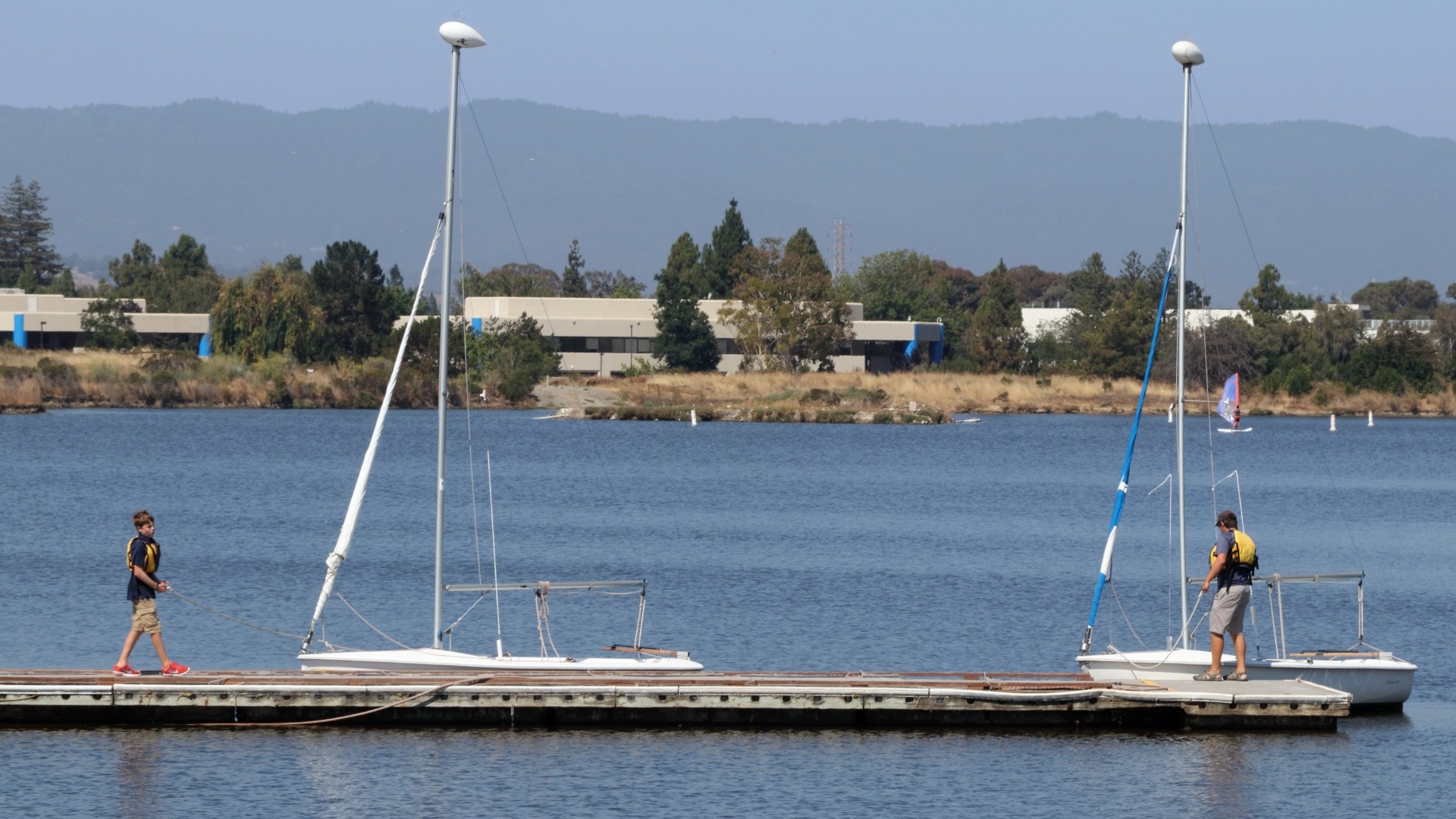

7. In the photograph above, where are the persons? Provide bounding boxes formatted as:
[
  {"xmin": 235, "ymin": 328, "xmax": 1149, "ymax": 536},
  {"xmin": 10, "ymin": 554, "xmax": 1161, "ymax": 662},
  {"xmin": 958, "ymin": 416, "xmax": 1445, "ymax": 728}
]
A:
[
  {"xmin": 1193, "ymin": 510, "xmax": 1257, "ymax": 681},
  {"xmin": 112, "ymin": 510, "xmax": 190, "ymax": 677},
  {"xmin": 1232, "ymin": 405, "xmax": 1241, "ymax": 431}
]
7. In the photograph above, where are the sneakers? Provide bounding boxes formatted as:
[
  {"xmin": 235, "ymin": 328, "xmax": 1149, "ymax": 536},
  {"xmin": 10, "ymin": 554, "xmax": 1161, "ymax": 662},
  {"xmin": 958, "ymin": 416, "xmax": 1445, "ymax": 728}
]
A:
[
  {"xmin": 113, "ymin": 664, "xmax": 141, "ymax": 677},
  {"xmin": 162, "ymin": 661, "xmax": 190, "ymax": 676}
]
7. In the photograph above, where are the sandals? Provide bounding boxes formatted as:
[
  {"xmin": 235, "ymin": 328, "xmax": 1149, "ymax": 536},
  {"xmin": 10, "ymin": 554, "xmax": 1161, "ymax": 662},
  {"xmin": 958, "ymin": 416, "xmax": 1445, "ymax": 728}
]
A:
[
  {"xmin": 1223, "ymin": 671, "xmax": 1248, "ymax": 681},
  {"xmin": 1193, "ymin": 669, "xmax": 1223, "ymax": 682}
]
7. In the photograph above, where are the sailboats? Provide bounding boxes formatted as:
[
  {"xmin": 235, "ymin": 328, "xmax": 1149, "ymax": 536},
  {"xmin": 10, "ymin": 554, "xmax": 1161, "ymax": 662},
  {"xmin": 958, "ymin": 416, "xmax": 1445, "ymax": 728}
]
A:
[
  {"xmin": 1216, "ymin": 372, "xmax": 1253, "ymax": 433},
  {"xmin": 1065, "ymin": 39, "xmax": 1420, "ymax": 706},
  {"xmin": 297, "ymin": 21, "xmax": 704, "ymax": 674}
]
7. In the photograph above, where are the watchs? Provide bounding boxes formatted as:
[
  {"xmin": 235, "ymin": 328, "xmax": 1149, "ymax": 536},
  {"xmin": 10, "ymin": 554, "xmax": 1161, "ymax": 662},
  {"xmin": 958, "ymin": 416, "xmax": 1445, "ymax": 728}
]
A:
[{"xmin": 154, "ymin": 582, "xmax": 158, "ymax": 590}]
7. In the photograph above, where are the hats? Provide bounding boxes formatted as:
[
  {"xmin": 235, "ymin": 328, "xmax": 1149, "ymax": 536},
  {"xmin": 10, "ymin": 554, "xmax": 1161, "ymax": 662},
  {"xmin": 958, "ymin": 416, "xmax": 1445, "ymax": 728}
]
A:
[{"xmin": 1215, "ymin": 510, "xmax": 1237, "ymax": 526}]
